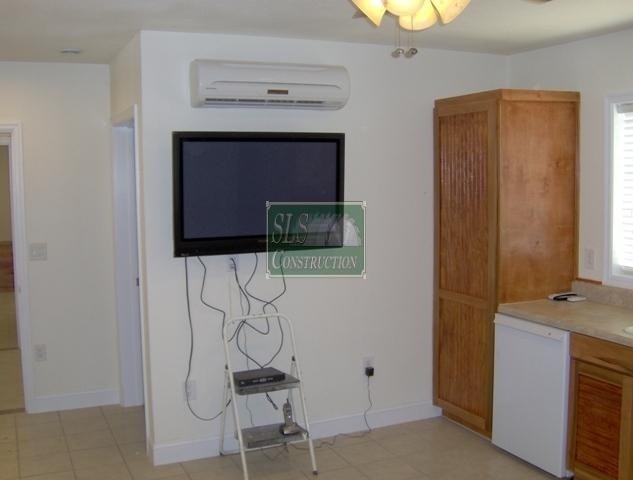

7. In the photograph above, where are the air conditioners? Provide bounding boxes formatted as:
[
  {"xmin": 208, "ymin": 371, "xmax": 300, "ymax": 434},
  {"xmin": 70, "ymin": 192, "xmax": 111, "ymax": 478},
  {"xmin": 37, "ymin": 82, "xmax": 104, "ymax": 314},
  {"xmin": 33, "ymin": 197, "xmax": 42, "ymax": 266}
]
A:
[{"xmin": 188, "ymin": 58, "xmax": 351, "ymax": 110}]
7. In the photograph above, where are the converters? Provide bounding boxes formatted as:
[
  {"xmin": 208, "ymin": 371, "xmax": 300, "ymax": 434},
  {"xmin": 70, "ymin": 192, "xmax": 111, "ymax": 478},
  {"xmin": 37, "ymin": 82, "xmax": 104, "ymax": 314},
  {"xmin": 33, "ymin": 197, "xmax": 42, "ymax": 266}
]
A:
[{"xmin": 366, "ymin": 367, "xmax": 374, "ymax": 376}]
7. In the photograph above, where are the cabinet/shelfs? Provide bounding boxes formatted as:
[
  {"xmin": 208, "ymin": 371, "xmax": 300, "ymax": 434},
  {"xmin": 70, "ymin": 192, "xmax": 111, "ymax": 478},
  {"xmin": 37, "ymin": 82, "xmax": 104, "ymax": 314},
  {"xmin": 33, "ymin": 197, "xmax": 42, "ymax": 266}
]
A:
[
  {"xmin": 218, "ymin": 314, "xmax": 318, "ymax": 480},
  {"xmin": 564, "ymin": 334, "xmax": 632, "ymax": 480},
  {"xmin": 429, "ymin": 85, "xmax": 582, "ymax": 444}
]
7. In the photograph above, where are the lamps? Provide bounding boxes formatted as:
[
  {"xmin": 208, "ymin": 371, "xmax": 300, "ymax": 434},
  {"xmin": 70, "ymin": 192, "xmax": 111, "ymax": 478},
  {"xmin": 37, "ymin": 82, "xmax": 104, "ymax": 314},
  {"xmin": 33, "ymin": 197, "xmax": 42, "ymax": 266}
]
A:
[{"xmin": 350, "ymin": 0, "xmax": 471, "ymax": 57}]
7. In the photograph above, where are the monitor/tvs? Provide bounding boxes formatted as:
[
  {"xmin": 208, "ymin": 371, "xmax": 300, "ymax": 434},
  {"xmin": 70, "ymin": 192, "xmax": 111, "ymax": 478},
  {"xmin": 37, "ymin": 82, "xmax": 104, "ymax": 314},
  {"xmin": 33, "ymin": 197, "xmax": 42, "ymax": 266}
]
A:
[{"xmin": 173, "ymin": 131, "xmax": 345, "ymax": 258}]
